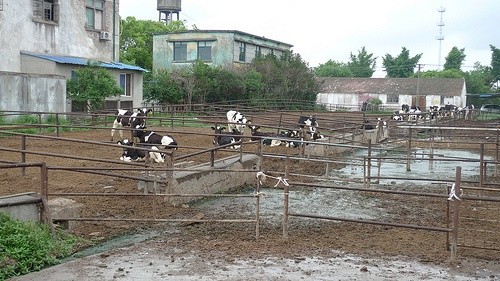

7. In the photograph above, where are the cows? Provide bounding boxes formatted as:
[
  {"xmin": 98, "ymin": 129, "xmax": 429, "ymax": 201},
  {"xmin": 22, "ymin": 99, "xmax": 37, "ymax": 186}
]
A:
[
  {"xmin": 211, "ymin": 110, "xmax": 324, "ymax": 150},
  {"xmin": 111, "ymin": 108, "xmax": 178, "ymax": 163},
  {"xmin": 375, "ymin": 104, "xmax": 474, "ymax": 128}
]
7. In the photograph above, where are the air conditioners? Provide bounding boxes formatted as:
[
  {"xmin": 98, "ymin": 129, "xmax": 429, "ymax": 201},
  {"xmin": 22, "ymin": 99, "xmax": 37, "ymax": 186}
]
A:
[{"xmin": 99, "ymin": 31, "xmax": 113, "ymax": 41}]
